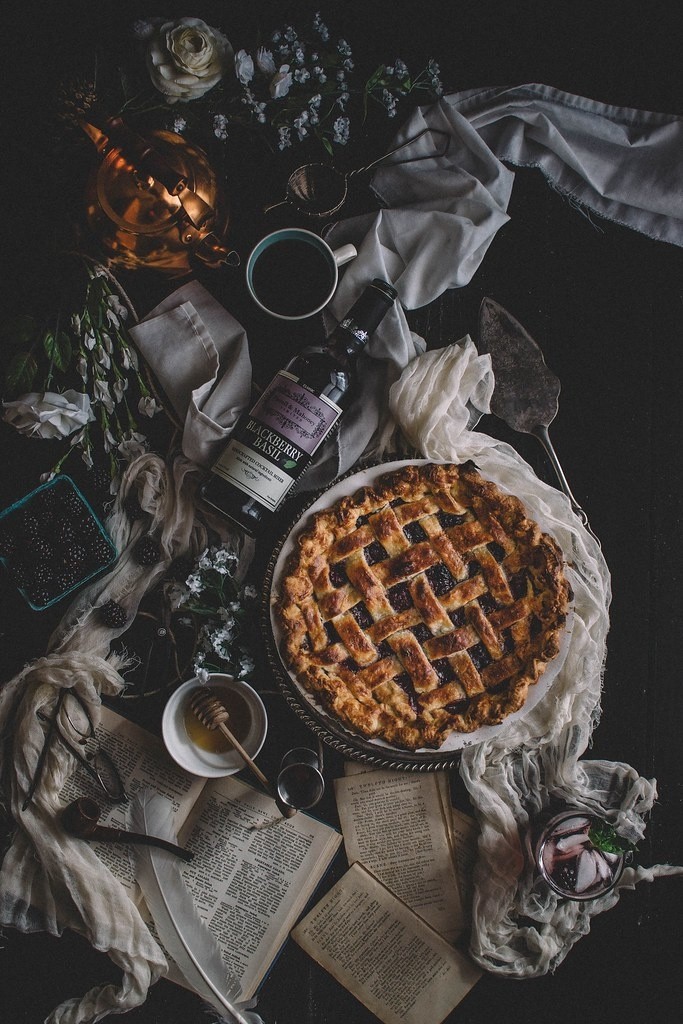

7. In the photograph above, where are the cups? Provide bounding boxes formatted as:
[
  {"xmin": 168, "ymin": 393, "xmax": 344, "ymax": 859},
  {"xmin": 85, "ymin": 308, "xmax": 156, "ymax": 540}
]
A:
[
  {"xmin": 246, "ymin": 229, "xmax": 357, "ymax": 320},
  {"xmin": 275, "ymin": 747, "xmax": 325, "ymax": 810},
  {"xmin": 161, "ymin": 673, "xmax": 268, "ymax": 778}
]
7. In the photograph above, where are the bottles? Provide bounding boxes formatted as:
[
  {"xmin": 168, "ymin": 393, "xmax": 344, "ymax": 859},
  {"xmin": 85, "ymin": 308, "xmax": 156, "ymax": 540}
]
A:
[{"xmin": 192, "ymin": 278, "xmax": 398, "ymax": 544}]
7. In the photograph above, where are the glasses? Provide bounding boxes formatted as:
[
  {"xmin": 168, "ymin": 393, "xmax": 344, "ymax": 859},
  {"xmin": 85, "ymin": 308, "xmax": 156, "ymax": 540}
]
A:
[{"xmin": 19, "ymin": 686, "xmax": 127, "ymax": 813}]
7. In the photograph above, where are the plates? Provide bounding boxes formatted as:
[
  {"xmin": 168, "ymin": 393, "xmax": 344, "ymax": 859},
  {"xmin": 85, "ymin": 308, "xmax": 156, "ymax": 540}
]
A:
[{"xmin": 261, "ymin": 454, "xmax": 462, "ymax": 773}]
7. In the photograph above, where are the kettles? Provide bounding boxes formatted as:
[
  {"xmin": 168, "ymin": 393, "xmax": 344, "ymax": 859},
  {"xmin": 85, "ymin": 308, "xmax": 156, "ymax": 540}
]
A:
[{"xmin": 75, "ymin": 117, "xmax": 242, "ymax": 276}]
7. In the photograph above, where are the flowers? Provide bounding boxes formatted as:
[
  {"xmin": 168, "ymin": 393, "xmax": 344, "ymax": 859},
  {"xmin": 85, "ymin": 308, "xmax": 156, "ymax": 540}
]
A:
[
  {"xmin": 0, "ymin": 257, "xmax": 176, "ymax": 489},
  {"xmin": 111, "ymin": 6, "xmax": 450, "ymax": 162},
  {"xmin": 166, "ymin": 547, "xmax": 260, "ymax": 686}
]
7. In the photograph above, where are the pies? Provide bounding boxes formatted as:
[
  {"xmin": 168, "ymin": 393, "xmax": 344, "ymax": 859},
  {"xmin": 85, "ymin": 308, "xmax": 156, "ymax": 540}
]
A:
[{"xmin": 270, "ymin": 462, "xmax": 574, "ymax": 753}]
